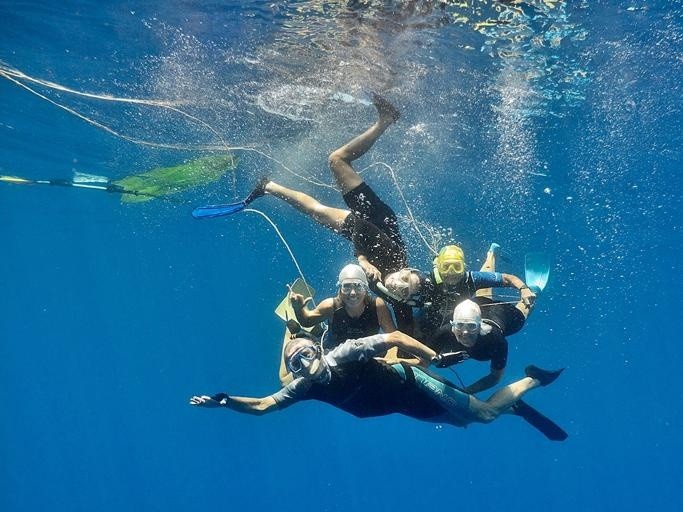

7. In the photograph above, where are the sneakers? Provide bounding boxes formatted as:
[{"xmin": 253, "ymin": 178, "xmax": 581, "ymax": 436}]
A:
[
  {"xmin": 489, "ymin": 243, "xmax": 501, "ymax": 254},
  {"xmin": 525, "ymin": 365, "xmax": 554, "ymax": 388},
  {"xmin": 513, "ymin": 400, "xmax": 533, "ymax": 416},
  {"xmin": 528, "ymin": 286, "xmax": 541, "ymax": 297},
  {"xmin": 244, "ymin": 176, "xmax": 269, "ymax": 205},
  {"xmin": 370, "ymin": 93, "xmax": 401, "ymax": 121}
]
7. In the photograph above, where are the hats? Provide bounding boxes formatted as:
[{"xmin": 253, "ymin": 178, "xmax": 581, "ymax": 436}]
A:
[
  {"xmin": 285, "ymin": 338, "xmax": 317, "ymax": 373},
  {"xmin": 453, "ymin": 300, "xmax": 481, "ymax": 322},
  {"xmin": 339, "ymin": 264, "xmax": 366, "ymax": 284},
  {"xmin": 437, "ymin": 246, "xmax": 465, "ymax": 263},
  {"xmin": 407, "ymin": 268, "xmax": 426, "ymax": 297}
]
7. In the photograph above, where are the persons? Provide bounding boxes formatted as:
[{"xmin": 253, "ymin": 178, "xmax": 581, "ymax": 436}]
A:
[
  {"xmin": 402, "ymin": 241, "xmax": 540, "ymax": 354},
  {"xmin": 372, "ymin": 241, "xmax": 551, "ymax": 397},
  {"xmin": 241, "ymin": 92, "xmax": 435, "ymax": 336},
  {"xmin": 271, "ymin": 261, "xmax": 398, "ymax": 389},
  {"xmin": 187, "ymin": 328, "xmax": 568, "ymax": 443}
]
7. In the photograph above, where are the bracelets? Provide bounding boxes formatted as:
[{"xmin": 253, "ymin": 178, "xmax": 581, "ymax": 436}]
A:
[
  {"xmin": 431, "ymin": 352, "xmax": 438, "ymax": 365},
  {"xmin": 519, "ymin": 285, "xmax": 530, "ymax": 292}
]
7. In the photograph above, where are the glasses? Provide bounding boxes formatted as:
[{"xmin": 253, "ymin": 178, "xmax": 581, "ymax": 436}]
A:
[
  {"xmin": 438, "ymin": 260, "xmax": 464, "ymax": 273},
  {"xmin": 452, "ymin": 322, "xmax": 480, "ymax": 332},
  {"xmin": 288, "ymin": 346, "xmax": 318, "ymax": 373},
  {"xmin": 340, "ymin": 283, "xmax": 366, "ymax": 291},
  {"xmin": 400, "ymin": 272, "xmax": 410, "ymax": 299}
]
